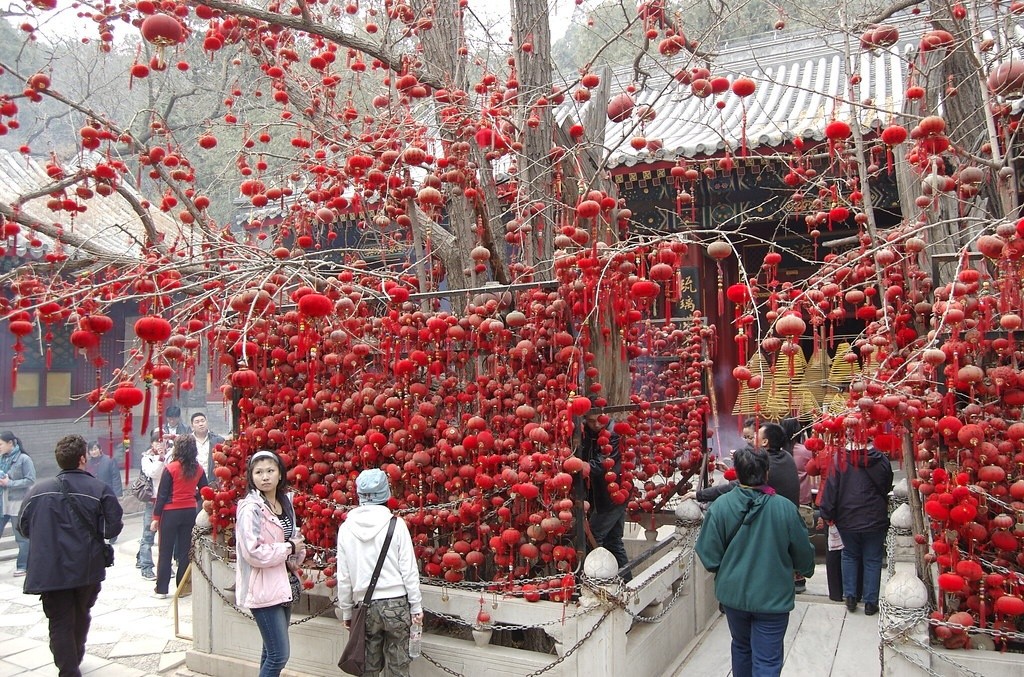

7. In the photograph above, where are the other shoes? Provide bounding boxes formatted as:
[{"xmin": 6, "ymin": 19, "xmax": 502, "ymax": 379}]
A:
[
  {"xmin": 856, "ymin": 597, "xmax": 863, "ymax": 601},
  {"xmin": 864, "ymin": 603, "xmax": 879, "ymax": 615},
  {"xmin": 179, "ymin": 585, "xmax": 192, "ymax": 597},
  {"xmin": 136, "ymin": 559, "xmax": 155, "ymax": 567},
  {"xmin": 142, "ymin": 571, "xmax": 157, "ymax": 581},
  {"xmin": 14, "ymin": 569, "xmax": 25, "ymax": 577},
  {"xmin": 831, "ymin": 595, "xmax": 843, "ymax": 601},
  {"xmin": 845, "ymin": 597, "xmax": 858, "ymax": 610},
  {"xmin": 171, "ymin": 569, "xmax": 175, "ymax": 578},
  {"xmin": 511, "ymin": 627, "xmax": 527, "ymax": 646},
  {"xmin": 794, "ymin": 582, "xmax": 806, "ymax": 593},
  {"xmin": 145, "ymin": 589, "xmax": 166, "ymax": 599}
]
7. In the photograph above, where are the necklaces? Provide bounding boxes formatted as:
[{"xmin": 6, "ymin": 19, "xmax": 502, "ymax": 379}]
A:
[{"xmin": 267, "ymin": 497, "xmax": 278, "ymax": 511}]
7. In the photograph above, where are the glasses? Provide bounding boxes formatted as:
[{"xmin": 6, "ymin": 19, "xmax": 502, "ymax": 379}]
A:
[{"xmin": 740, "ymin": 435, "xmax": 756, "ymax": 444}]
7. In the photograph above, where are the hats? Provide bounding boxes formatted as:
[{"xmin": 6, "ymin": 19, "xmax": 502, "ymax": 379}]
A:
[
  {"xmin": 355, "ymin": 468, "xmax": 391, "ymax": 506},
  {"xmin": 151, "ymin": 427, "xmax": 168, "ymax": 440}
]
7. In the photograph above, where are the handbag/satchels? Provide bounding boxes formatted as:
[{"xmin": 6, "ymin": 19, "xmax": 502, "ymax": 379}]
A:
[
  {"xmin": 337, "ymin": 606, "xmax": 366, "ymax": 676},
  {"xmin": 281, "ymin": 576, "xmax": 300, "ymax": 607},
  {"xmin": 103, "ymin": 544, "xmax": 115, "ymax": 567},
  {"xmin": 131, "ymin": 458, "xmax": 153, "ymax": 502},
  {"xmin": 797, "ymin": 506, "xmax": 815, "ymax": 527}
]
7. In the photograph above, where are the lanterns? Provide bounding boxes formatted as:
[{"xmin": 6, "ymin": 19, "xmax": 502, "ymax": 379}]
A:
[
  {"xmin": 209, "ymin": 190, "xmax": 715, "ymax": 628},
  {"xmin": 706, "ymin": 0, "xmax": 1024, "ymax": 653},
  {"xmin": 0, "ymin": 1, "xmax": 723, "ymax": 205},
  {"xmin": 1, "ymin": 186, "xmax": 250, "ymax": 486}
]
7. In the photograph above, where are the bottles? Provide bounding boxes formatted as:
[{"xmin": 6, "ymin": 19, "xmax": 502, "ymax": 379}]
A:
[
  {"xmin": 0, "ymin": 471, "xmax": 7, "ymax": 479},
  {"xmin": 409, "ymin": 624, "xmax": 422, "ymax": 657},
  {"xmin": 289, "ymin": 527, "xmax": 301, "ymax": 563}
]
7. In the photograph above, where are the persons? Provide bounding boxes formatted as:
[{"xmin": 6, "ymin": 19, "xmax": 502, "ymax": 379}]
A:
[
  {"xmin": 16, "ymin": 435, "xmax": 123, "ymax": 677},
  {"xmin": 336, "ymin": 468, "xmax": 424, "ymax": 677},
  {"xmin": 135, "ymin": 405, "xmax": 228, "ymax": 599},
  {"xmin": 0, "ymin": 430, "xmax": 36, "ymax": 578},
  {"xmin": 582, "ymin": 395, "xmax": 633, "ymax": 587},
  {"xmin": 235, "ymin": 449, "xmax": 307, "ymax": 677},
  {"xmin": 679, "ymin": 415, "xmax": 895, "ymax": 677},
  {"xmin": 85, "ymin": 440, "xmax": 123, "ymax": 546}
]
